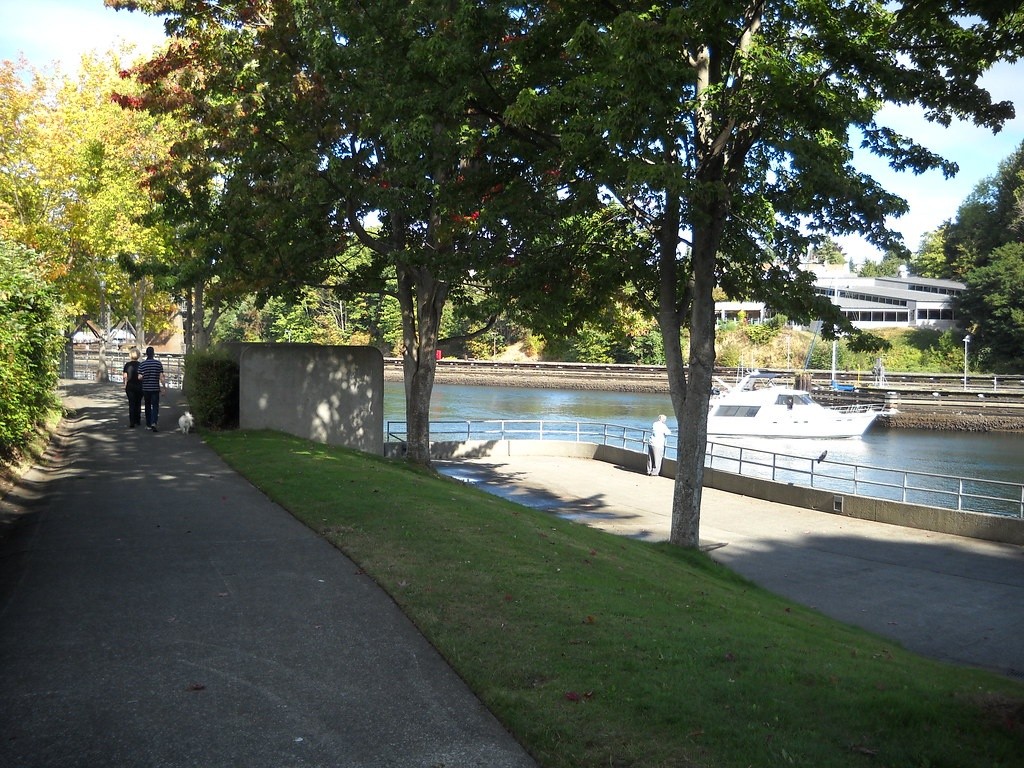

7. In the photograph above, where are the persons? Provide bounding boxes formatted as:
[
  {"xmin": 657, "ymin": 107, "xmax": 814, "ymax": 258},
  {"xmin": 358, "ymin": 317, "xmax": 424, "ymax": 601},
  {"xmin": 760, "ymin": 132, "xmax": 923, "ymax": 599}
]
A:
[
  {"xmin": 137, "ymin": 347, "xmax": 166, "ymax": 432},
  {"xmin": 646, "ymin": 415, "xmax": 671, "ymax": 476},
  {"xmin": 123, "ymin": 349, "xmax": 142, "ymax": 429}
]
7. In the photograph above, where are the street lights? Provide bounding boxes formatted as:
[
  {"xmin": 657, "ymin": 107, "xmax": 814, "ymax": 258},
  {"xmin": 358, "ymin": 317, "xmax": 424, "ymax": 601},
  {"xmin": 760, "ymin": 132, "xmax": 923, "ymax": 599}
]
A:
[
  {"xmin": 285, "ymin": 331, "xmax": 290, "ymax": 342},
  {"xmin": 491, "ymin": 333, "xmax": 496, "ymax": 362},
  {"xmin": 99, "ymin": 280, "xmax": 108, "ymax": 383},
  {"xmin": 964, "ymin": 335, "xmax": 969, "ymax": 392}
]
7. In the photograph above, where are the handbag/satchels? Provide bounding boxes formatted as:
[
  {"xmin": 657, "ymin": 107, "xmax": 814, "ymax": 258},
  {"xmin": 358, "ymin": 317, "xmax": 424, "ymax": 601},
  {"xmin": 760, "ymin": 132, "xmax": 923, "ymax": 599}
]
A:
[{"xmin": 127, "ymin": 362, "xmax": 133, "ymax": 380}]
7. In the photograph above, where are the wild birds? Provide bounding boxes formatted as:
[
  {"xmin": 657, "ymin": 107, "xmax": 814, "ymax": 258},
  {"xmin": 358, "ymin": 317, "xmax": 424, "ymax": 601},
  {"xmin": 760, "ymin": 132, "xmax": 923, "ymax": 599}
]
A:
[{"xmin": 817, "ymin": 450, "xmax": 828, "ymax": 464}]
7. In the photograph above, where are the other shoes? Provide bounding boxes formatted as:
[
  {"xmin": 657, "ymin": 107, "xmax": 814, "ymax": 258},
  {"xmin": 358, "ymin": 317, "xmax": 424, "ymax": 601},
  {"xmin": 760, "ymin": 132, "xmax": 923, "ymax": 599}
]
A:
[
  {"xmin": 130, "ymin": 425, "xmax": 135, "ymax": 428},
  {"xmin": 134, "ymin": 421, "xmax": 140, "ymax": 425}
]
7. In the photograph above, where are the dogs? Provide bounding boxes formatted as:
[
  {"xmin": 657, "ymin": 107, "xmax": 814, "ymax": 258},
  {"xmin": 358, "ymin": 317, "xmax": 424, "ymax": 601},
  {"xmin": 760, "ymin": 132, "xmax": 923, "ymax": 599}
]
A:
[{"xmin": 178, "ymin": 411, "xmax": 194, "ymax": 435}]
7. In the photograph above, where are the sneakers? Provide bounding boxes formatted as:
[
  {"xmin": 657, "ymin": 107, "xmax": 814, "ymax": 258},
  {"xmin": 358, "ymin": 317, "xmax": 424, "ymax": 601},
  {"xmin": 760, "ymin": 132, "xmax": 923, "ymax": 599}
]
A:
[
  {"xmin": 151, "ymin": 423, "xmax": 158, "ymax": 432},
  {"xmin": 145, "ymin": 425, "xmax": 152, "ymax": 430}
]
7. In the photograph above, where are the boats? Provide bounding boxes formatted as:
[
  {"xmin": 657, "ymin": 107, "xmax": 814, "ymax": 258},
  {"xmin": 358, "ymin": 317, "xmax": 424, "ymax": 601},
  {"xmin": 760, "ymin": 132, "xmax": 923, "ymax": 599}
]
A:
[{"xmin": 707, "ymin": 338, "xmax": 898, "ymax": 439}]
